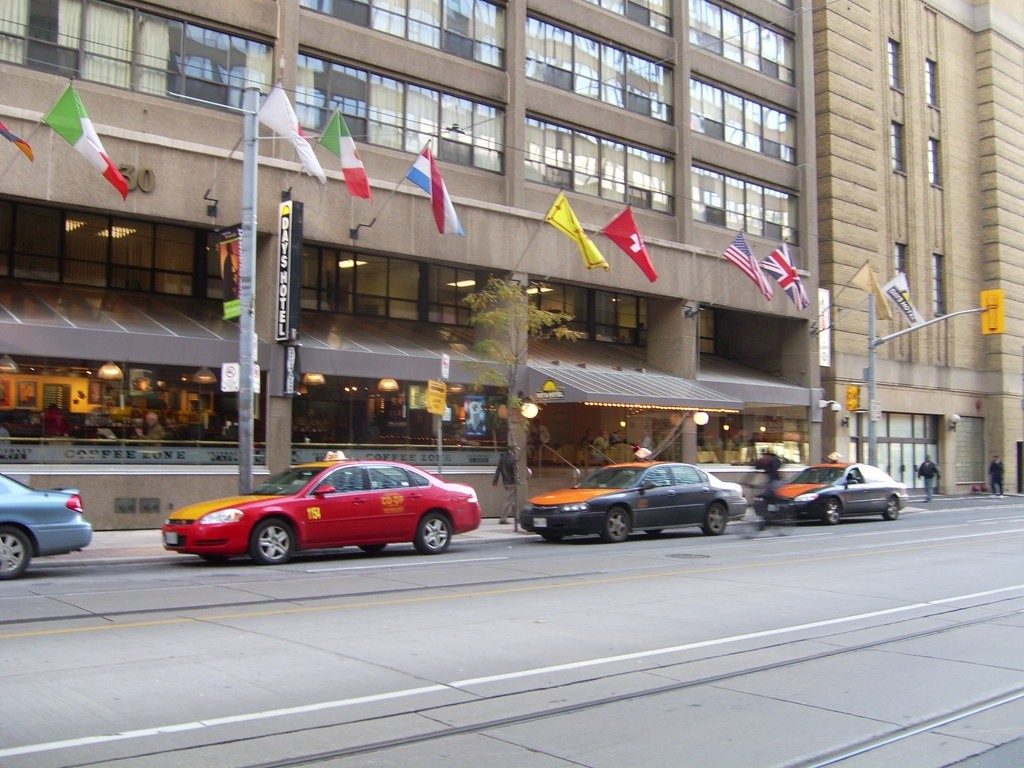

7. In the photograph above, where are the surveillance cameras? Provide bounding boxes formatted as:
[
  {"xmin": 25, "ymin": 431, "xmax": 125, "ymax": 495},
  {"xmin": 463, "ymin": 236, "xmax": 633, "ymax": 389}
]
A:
[{"xmin": 831, "ymin": 404, "xmax": 841, "ymax": 412}]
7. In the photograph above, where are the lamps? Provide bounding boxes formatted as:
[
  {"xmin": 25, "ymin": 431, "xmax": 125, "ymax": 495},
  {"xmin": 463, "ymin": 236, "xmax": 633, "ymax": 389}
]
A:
[
  {"xmin": 192, "ymin": 367, "xmax": 217, "ymax": 383},
  {"xmin": 97, "ymin": 361, "xmax": 124, "ymax": 380},
  {"xmin": 0, "ymin": 354, "xmax": 20, "ymax": 375},
  {"xmin": 692, "ymin": 408, "xmax": 710, "ymax": 426},
  {"xmin": 302, "ymin": 373, "xmax": 326, "ymax": 386},
  {"xmin": 516, "ymin": 390, "xmax": 538, "ymax": 419},
  {"xmin": 377, "ymin": 378, "xmax": 400, "ymax": 391},
  {"xmin": 949, "ymin": 413, "xmax": 960, "ymax": 432}
]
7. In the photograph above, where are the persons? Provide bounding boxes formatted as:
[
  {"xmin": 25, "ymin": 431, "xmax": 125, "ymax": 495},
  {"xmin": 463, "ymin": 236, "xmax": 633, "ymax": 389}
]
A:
[
  {"xmin": 578, "ymin": 428, "xmax": 628, "ymax": 468},
  {"xmin": 917, "ymin": 453, "xmax": 941, "ymax": 503},
  {"xmin": 987, "ymin": 456, "xmax": 1005, "ymax": 498},
  {"xmin": 445, "ymin": 423, "xmax": 481, "ymax": 447},
  {"xmin": 42, "ymin": 399, "xmax": 206, "ymax": 446},
  {"xmin": 757, "ymin": 447, "xmax": 782, "ymax": 489},
  {"xmin": 491, "ymin": 441, "xmax": 521, "ymax": 524}
]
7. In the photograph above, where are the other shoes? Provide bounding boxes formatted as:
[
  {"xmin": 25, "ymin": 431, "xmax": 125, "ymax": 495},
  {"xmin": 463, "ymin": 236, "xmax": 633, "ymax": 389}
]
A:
[
  {"xmin": 998, "ymin": 494, "xmax": 1003, "ymax": 498},
  {"xmin": 989, "ymin": 494, "xmax": 996, "ymax": 498},
  {"xmin": 925, "ymin": 498, "xmax": 931, "ymax": 503},
  {"xmin": 499, "ymin": 520, "xmax": 510, "ymax": 524}
]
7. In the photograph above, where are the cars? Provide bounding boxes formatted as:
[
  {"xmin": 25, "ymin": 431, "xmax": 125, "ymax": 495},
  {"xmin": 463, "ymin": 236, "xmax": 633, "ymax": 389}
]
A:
[
  {"xmin": 161, "ymin": 451, "xmax": 483, "ymax": 573},
  {"xmin": 521, "ymin": 462, "xmax": 749, "ymax": 543},
  {"xmin": 0, "ymin": 472, "xmax": 94, "ymax": 578},
  {"xmin": 753, "ymin": 462, "xmax": 909, "ymax": 524}
]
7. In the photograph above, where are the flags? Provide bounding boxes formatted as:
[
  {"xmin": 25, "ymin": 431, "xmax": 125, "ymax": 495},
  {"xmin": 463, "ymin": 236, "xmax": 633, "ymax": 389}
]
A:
[
  {"xmin": 256, "ymin": 82, "xmax": 328, "ymax": 186},
  {"xmin": 759, "ymin": 241, "xmax": 811, "ymax": 311},
  {"xmin": 44, "ymin": 85, "xmax": 129, "ymax": 200},
  {"xmin": 0, "ymin": 120, "xmax": 34, "ymax": 163},
  {"xmin": 317, "ymin": 107, "xmax": 371, "ymax": 198},
  {"xmin": 882, "ymin": 271, "xmax": 926, "ymax": 328},
  {"xmin": 721, "ymin": 231, "xmax": 773, "ymax": 302},
  {"xmin": 850, "ymin": 262, "xmax": 892, "ymax": 321},
  {"xmin": 405, "ymin": 144, "xmax": 465, "ymax": 237},
  {"xmin": 543, "ymin": 191, "xmax": 609, "ymax": 273},
  {"xmin": 600, "ymin": 205, "xmax": 657, "ymax": 283}
]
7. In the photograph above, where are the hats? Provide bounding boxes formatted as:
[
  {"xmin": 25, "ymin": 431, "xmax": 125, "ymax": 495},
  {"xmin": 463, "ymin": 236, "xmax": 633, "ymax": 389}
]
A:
[
  {"xmin": 759, "ymin": 447, "xmax": 777, "ymax": 456},
  {"xmin": 505, "ymin": 442, "xmax": 520, "ymax": 451},
  {"xmin": 925, "ymin": 454, "xmax": 930, "ymax": 459}
]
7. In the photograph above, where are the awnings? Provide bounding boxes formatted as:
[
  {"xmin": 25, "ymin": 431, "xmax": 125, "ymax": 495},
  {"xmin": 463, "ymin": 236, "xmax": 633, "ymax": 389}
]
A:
[{"xmin": 528, "ymin": 366, "xmax": 745, "ymax": 415}]
